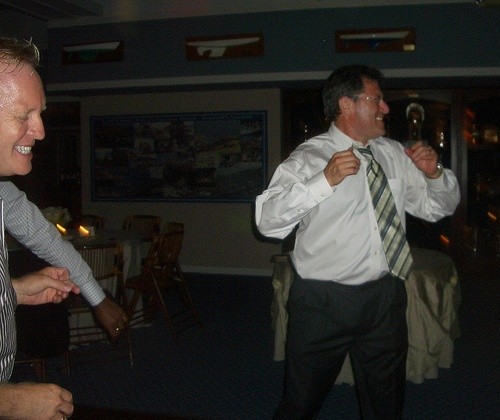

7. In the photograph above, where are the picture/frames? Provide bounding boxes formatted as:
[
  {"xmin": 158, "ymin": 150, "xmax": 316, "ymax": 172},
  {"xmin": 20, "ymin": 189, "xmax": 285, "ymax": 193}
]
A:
[{"xmin": 88, "ymin": 109, "xmax": 269, "ymax": 203}]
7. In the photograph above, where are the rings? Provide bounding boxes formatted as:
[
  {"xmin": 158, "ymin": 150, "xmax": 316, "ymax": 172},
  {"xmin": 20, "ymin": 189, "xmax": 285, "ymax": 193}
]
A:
[
  {"xmin": 116, "ymin": 328, "xmax": 119, "ymax": 330},
  {"xmin": 62, "ymin": 415, "xmax": 65, "ymax": 420},
  {"xmin": 427, "ymin": 149, "xmax": 431, "ymax": 155}
]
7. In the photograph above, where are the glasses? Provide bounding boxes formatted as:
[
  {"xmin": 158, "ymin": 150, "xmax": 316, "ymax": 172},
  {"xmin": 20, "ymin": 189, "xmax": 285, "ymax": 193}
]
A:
[{"xmin": 358, "ymin": 94, "xmax": 383, "ymax": 104}]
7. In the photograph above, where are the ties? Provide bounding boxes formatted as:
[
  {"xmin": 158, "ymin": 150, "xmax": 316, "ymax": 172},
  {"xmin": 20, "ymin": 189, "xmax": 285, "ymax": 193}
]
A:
[{"xmin": 352, "ymin": 142, "xmax": 412, "ymax": 281}]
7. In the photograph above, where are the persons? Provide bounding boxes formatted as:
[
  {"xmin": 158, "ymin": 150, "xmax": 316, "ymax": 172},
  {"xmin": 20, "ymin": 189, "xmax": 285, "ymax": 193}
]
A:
[
  {"xmin": 0, "ymin": 181, "xmax": 127, "ymax": 340},
  {"xmin": 0, "ymin": 36, "xmax": 80, "ymax": 420},
  {"xmin": 254, "ymin": 64, "xmax": 461, "ymax": 420}
]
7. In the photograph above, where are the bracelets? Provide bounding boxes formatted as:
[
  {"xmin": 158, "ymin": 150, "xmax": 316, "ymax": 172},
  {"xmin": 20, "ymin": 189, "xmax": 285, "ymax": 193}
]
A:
[{"xmin": 428, "ymin": 163, "xmax": 442, "ymax": 179}]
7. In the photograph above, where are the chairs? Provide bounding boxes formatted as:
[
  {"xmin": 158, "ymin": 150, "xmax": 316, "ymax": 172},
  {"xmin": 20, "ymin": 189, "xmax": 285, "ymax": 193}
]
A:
[{"xmin": 11, "ymin": 214, "xmax": 203, "ymax": 382}]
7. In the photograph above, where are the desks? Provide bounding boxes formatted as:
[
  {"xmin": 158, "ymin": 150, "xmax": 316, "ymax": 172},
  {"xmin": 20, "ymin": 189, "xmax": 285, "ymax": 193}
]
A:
[
  {"xmin": 9, "ymin": 229, "xmax": 143, "ymax": 352},
  {"xmin": 272, "ymin": 246, "xmax": 455, "ymax": 387}
]
